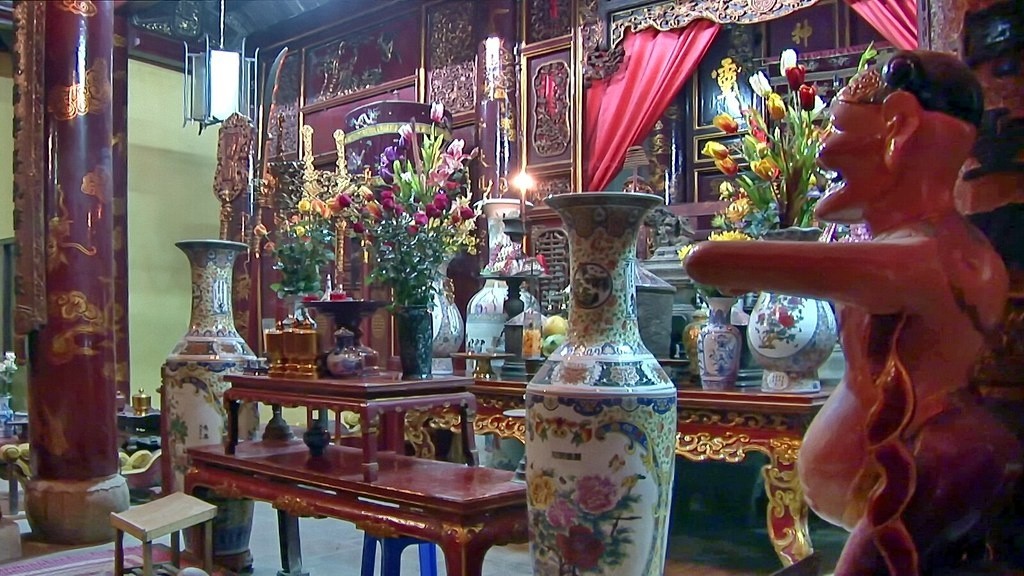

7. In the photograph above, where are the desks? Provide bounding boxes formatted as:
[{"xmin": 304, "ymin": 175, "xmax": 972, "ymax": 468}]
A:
[
  {"xmin": 184, "ymin": 435, "xmax": 527, "ymax": 576},
  {"xmin": 469, "ymin": 378, "xmax": 836, "ymax": 576},
  {"xmin": 220, "ymin": 373, "xmax": 483, "ymax": 484}
]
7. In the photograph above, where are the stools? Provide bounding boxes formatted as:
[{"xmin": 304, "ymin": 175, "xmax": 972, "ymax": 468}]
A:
[{"xmin": 359, "ymin": 529, "xmax": 438, "ymax": 576}]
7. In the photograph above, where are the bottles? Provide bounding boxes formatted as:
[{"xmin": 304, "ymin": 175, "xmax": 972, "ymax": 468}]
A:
[
  {"xmin": 303, "ymin": 419, "xmax": 330, "ymax": 457},
  {"xmin": 262, "ymin": 405, "xmax": 294, "ymax": 443},
  {"xmin": 325, "ymin": 326, "xmax": 363, "ymax": 378}
]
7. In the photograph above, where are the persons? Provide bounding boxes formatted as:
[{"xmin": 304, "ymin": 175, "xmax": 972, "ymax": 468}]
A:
[{"xmin": 683, "ymin": 52, "xmax": 1010, "ymax": 576}]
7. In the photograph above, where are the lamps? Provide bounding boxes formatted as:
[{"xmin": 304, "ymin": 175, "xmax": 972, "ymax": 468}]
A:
[{"xmin": 181, "ymin": 1, "xmax": 260, "ymax": 133}]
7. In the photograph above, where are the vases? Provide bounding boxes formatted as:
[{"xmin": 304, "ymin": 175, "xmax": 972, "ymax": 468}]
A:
[
  {"xmin": 747, "ymin": 224, "xmax": 836, "ymax": 397},
  {"xmin": 163, "ymin": 239, "xmax": 261, "ymax": 570},
  {"xmin": 697, "ymin": 294, "xmax": 742, "ymax": 391},
  {"xmin": 429, "ymin": 238, "xmax": 463, "ymax": 378},
  {"xmin": 525, "ymin": 191, "xmax": 676, "ymax": 576},
  {"xmin": 464, "ymin": 193, "xmax": 543, "ymax": 475},
  {"xmin": 284, "ymin": 293, "xmax": 310, "ymax": 323},
  {"xmin": 398, "ymin": 304, "xmax": 436, "ymax": 381}
]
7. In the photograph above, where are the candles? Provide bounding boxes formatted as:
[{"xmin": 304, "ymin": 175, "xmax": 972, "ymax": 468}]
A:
[{"xmin": 517, "ymin": 171, "xmax": 527, "ymax": 223}]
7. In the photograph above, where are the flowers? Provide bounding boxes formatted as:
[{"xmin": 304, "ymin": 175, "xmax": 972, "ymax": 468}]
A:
[
  {"xmin": 674, "ymin": 235, "xmax": 760, "ymax": 299},
  {"xmin": 250, "ymin": 102, "xmax": 478, "ymax": 312},
  {"xmin": 703, "ymin": 45, "xmax": 883, "ymax": 222}
]
